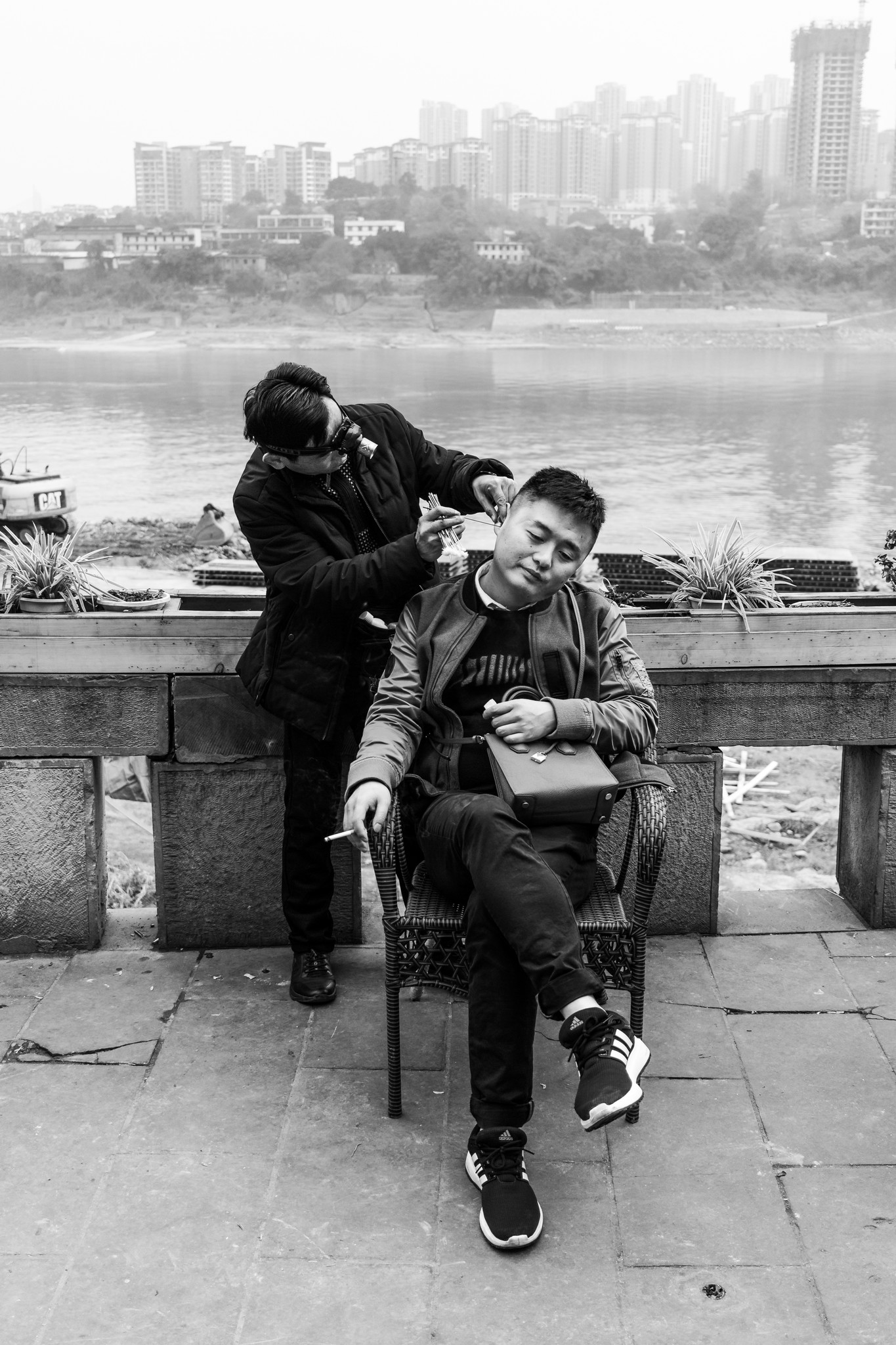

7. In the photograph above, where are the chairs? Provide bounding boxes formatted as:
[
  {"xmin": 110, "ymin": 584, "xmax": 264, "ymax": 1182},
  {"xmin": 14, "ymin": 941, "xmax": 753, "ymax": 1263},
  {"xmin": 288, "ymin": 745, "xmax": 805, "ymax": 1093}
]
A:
[{"xmin": 364, "ymin": 719, "xmax": 667, "ymax": 1123}]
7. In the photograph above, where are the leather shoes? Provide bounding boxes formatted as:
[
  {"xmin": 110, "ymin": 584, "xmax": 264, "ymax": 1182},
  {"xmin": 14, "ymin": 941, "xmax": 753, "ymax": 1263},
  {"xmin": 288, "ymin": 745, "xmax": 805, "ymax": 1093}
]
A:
[{"xmin": 288, "ymin": 945, "xmax": 340, "ymax": 1007}]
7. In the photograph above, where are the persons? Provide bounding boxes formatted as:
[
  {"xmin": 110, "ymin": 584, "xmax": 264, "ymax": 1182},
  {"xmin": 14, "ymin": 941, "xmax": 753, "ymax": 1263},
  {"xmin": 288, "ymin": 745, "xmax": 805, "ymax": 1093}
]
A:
[
  {"xmin": 233, "ymin": 362, "xmax": 517, "ymax": 1004},
  {"xmin": 342, "ymin": 467, "xmax": 660, "ymax": 1250}
]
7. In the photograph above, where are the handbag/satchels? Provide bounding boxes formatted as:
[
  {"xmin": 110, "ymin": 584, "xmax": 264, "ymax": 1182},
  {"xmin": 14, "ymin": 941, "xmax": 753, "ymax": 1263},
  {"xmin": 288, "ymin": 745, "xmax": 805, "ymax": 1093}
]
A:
[{"xmin": 483, "ymin": 683, "xmax": 620, "ymax": 823}]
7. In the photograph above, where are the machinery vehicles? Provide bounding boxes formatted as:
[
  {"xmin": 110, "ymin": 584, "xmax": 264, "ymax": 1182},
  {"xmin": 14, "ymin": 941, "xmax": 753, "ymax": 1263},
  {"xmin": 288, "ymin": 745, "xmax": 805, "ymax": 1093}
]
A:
[{"xmin": 0, "ymin": 446, "xmax": 77, "ymax": 546}]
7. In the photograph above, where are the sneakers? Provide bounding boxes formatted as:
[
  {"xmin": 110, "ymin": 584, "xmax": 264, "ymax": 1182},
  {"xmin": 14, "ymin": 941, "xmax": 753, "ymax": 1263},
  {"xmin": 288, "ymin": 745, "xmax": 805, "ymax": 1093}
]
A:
[
  {"xmin": 464, "ymin": 1120, "xmax": 544, "ymax": 1250},
  {"xmin": 558, "ymin": 1006, "xmax": 651, "ymax": 1135}
]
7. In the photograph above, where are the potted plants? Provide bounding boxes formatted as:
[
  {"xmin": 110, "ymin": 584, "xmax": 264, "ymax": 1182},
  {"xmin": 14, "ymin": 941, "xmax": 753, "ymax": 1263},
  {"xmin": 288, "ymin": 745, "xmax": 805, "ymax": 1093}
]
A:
[
  {"xmin": 0, "ymin": 520, "xmax": 114, "ymax": 614},
  {"xmin": 641, "ymin": 517, "xmax": 798, "ymax": 633}
]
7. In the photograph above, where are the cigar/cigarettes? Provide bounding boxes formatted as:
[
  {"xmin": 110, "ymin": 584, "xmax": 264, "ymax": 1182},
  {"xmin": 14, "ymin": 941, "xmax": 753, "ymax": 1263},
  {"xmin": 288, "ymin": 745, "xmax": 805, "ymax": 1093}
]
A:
[{"xmin": 324, "ymin": 826, "xmax": 367, "ymax": 842}]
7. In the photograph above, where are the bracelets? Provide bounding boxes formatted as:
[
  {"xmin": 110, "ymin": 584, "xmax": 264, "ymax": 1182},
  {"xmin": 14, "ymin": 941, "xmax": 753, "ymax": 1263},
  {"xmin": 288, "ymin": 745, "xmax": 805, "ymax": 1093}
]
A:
[{"xmin": 480, "ymin": 471, "xmax": 497, "ymax": 476}]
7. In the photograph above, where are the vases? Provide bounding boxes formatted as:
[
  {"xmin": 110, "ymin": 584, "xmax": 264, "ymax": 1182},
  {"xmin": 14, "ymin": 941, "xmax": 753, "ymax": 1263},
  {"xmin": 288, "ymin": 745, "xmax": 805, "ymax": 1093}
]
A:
[{"xmin": 97, "ymin": 590, "xmax": 170, "ymax": 612}]
